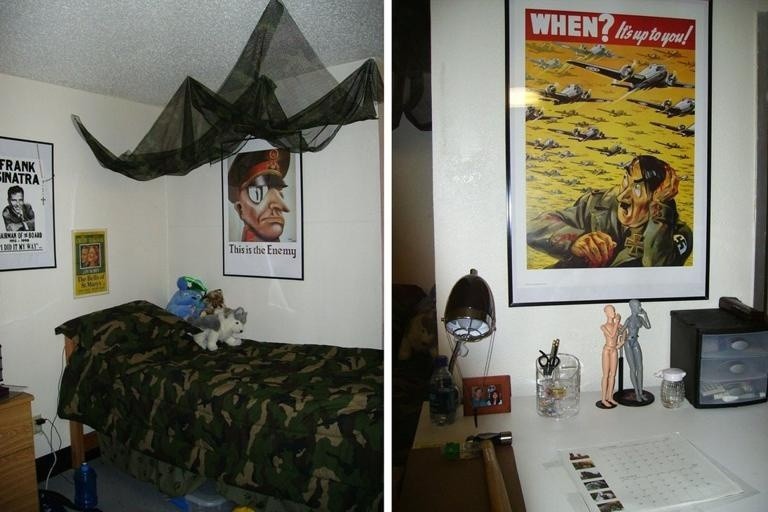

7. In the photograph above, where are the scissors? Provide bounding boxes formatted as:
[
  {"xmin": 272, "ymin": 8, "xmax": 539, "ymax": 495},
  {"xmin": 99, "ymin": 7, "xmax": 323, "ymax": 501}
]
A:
[{"xmin": 538, "ymin": 350, "xmax": 560, "ymax": 376}]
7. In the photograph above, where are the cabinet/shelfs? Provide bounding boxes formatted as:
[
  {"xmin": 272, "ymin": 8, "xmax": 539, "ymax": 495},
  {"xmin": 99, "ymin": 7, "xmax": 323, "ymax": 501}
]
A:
[{"xmin": 670, "ymin": 310, "xmax": 768, "ymax": 410}]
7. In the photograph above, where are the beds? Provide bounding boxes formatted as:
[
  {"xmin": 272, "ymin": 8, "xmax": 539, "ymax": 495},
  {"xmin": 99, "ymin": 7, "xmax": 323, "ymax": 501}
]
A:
[{"xmin": 64, "ymin": 300, "xmax": 384, "ymax": 512}]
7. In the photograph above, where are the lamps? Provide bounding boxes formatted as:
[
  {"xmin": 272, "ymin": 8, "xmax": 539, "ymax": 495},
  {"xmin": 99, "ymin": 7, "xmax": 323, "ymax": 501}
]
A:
[{"xmin": 429, "ymin": 268, "xmax": 496, "ymax": 427}]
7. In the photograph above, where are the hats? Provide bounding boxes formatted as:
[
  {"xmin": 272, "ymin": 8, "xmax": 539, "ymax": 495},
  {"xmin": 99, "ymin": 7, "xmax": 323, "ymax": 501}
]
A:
[{"xmin": 227, "ymin": 147, "xmax": 291, "ymax": 204}]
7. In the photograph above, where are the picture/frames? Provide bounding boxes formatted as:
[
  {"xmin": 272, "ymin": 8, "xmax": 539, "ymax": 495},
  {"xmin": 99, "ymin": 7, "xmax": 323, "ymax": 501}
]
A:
[
  {"xmin": 504, "ymin": 0, "xmax": 713, "ymax": 307},
  {"xmin": 219, "ymin": 130, "xmax": 304, "ymax": 281},
  {"xmin": 0, "ymin": 135, "xmax": 58, "ymax": 272}
]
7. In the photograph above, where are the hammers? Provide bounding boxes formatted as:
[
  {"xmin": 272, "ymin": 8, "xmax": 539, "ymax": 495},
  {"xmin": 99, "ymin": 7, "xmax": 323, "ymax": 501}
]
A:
[{"xmin": 465, "ymin": 432, "xmax": 513, "ymax": 512}]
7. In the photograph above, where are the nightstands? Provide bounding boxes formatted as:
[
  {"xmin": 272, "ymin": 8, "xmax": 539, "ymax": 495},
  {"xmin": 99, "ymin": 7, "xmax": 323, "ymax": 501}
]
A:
[{"xmin": 0, "ymin": 390, "xmax": 41, "ymax": 512}]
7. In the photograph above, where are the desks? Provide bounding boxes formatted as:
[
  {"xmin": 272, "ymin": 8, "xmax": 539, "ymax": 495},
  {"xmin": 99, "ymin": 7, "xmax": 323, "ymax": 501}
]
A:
[{"xmin": 399, "ymin": 388, "xmax": 768, "ymax": 512}]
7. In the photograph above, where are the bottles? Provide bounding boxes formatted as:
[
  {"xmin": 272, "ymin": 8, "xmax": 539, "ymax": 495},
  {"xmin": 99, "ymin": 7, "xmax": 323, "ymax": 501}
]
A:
[
  {"xmin": 662, "ymin": 369, "xmax": 685, "ymax": 408},
  {"xmin": 430, "ymin": 356, "xmax": 455, "ymax": 426}
]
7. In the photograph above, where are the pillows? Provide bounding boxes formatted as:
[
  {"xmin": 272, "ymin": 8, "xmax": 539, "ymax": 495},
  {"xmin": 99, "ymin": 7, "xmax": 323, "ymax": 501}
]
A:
[{"xmin": 55, "ymin": 300, "xmax": 201, "ymax": 360}]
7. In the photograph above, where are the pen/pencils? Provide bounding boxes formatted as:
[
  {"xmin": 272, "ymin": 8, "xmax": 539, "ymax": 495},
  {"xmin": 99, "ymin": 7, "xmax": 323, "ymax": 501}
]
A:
[
  {"xmin": 550, "ymin": 339, "xmax": 555, "ymax": 366},
  {"xmin": 556, "ymin": 338, "xmax": 560, "ymax": 366}
]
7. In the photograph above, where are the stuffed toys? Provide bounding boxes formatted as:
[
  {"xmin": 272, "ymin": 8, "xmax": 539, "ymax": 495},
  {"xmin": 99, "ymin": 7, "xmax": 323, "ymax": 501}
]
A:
[
  {"xmin": 165, "ymin": 276, "xmax": 204, "ymax": 316},
  {"xmin": 190, "ymin": 306, "xmax": 247, "ymax": 353}
]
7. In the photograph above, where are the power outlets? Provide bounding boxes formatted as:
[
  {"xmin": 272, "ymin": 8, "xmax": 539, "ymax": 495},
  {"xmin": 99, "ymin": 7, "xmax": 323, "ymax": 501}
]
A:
[{"xmin": 30, "ymin": 415, "xmax": 43, "ymax": 434}]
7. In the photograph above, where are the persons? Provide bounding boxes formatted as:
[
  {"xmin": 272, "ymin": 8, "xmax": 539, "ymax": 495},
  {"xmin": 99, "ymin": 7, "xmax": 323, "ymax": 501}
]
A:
[
  {"xmin": 486, "ymin": 390, "xmax": 504, "ymax": 407},
  {"xmin": 618, "ymin": 300, "xmax": 652, "ymax": 402},
  {"xmin": 472, "ymin": 388, "xmax": 488, "ymax": 407},
  {"xmin": 601, "ymin": 306, "xmax": 627, "ymax": 407}
]
